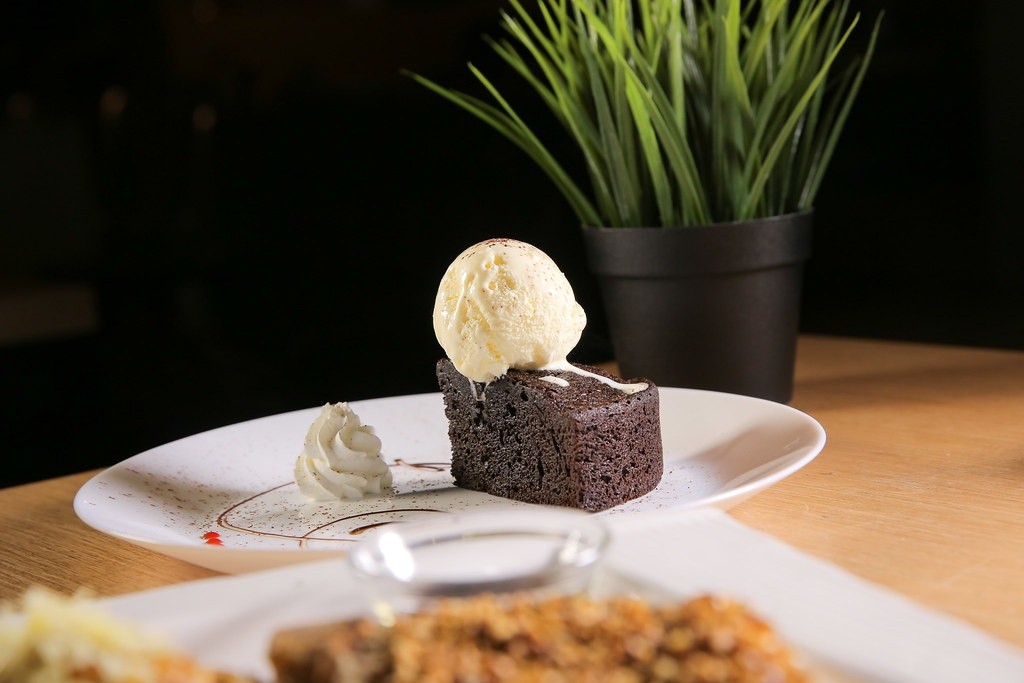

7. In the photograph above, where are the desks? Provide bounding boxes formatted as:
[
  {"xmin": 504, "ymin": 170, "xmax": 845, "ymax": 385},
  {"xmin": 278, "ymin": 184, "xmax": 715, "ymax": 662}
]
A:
[{"xmin": 0, "ymin": 333, "xmax": 1024, "ymax": 649}]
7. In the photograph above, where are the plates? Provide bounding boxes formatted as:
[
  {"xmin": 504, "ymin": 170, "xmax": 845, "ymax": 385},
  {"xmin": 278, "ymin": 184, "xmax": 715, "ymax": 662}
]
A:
[
  {"xmin": 71, "ymin": 381, "xmax": 828, "ymax": 577},
  {"xmin": 0, "ymin": 510, "xmax": 1024, "ymax": 683}
]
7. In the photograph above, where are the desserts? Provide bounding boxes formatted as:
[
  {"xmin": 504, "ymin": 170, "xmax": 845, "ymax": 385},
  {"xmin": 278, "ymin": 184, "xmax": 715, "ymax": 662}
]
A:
[
  {"xmin": 293, "ymin": 400, "xmax": 393, "ymax": 503},
  {"xmin": 432, "ymin": 237, "xmax": 664, "ymax": 513}
]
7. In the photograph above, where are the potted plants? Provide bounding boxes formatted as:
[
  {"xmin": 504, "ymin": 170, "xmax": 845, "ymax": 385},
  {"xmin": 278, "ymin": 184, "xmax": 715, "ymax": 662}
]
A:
[{"xmin": 403, "ymin": 0, "xmax": 884, "ymax": 406}]
